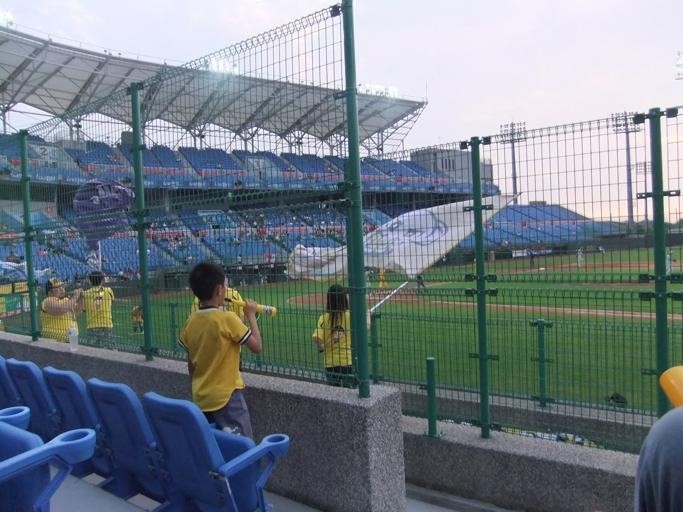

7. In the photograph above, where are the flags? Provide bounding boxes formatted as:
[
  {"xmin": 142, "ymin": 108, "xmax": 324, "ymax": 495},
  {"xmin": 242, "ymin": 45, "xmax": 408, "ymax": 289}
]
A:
[{"xmin": 291, "ymin": 192, "xmax": 523, "ymax": 290}]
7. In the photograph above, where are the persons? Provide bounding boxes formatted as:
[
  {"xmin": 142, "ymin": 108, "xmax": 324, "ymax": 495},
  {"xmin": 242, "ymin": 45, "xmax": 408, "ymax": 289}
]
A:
[
  {"xmin": 131, "ymin": 304, "xmax": 144, "ymax": 334},
  {"xmin": 1, "ymin": 156, "xmax": 438, "ymax": 290},
  {"xmin": 527, "ymin": 248, "xmax": 536, "ymax": 269},
  {"xmin": 177, "ymin": 262, "xmax": 262, "ymax": 439},
  {"xmin": 40, "ymin": 277, "xmax": 85, "ymax": 342},
  {"xmin": 665, "ymin": 250, "xmax": 673, "ymax": 274},
  {"xmin": 576, "ymin": 248, "xmax": 585, "ymax": 268},
  {"xmin": 312, "ymin": 284, "xmax": 376, "ymax": 388},
  {"xmin": 190, "ymin": 287, "xmax": 248, "ymax": 371},
  {"xmin": 83, "ymin": 271, "xmax": 118, "ymax": 351}
]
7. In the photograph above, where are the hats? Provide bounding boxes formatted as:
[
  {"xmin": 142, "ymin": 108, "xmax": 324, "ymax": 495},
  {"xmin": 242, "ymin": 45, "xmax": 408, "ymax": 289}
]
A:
[{"xmin": 45, "ymin": 277, "xmax": 65, "ymax": 289}]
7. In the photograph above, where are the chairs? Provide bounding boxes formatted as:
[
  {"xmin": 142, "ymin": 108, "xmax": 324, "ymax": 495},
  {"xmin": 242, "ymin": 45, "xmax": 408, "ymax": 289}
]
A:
[
  {"xmin": 0, "ymin": 407, "xmax": 97, "ymax": 512},
  {"xmin": 0, "ymin": 356, "xmax": 23, "ymax": 409},
  {"xmin": 42, "ymin": 365, "xmax": 132, "ymax": 501},
  {"xmin": 6, "ymin": 358, "xmax": 92, "ymax": 479},
  {"xmin": 142, "ymin": 391, "xmax": 290, "ymax": 512},
  {"xmin": 86, "ymin": 377, "xmax": 170, "ymax": 512}
]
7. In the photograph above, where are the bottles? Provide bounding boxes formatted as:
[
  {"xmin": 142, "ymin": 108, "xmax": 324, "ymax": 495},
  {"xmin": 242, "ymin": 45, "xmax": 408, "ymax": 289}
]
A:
[{"xmin": 69, "ymin": 324, "xmax": 78, "ymax": 351}]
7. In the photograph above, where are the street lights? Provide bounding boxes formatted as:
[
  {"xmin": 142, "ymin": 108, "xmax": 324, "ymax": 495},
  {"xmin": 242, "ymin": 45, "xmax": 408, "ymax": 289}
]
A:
[
  {"xmin": 611, "ymin": 110, "xmax": 644, "ymax": 222},
  {"xmin": 497, "ymin": 121, "xmax": 529, "ymax": 204}
]
